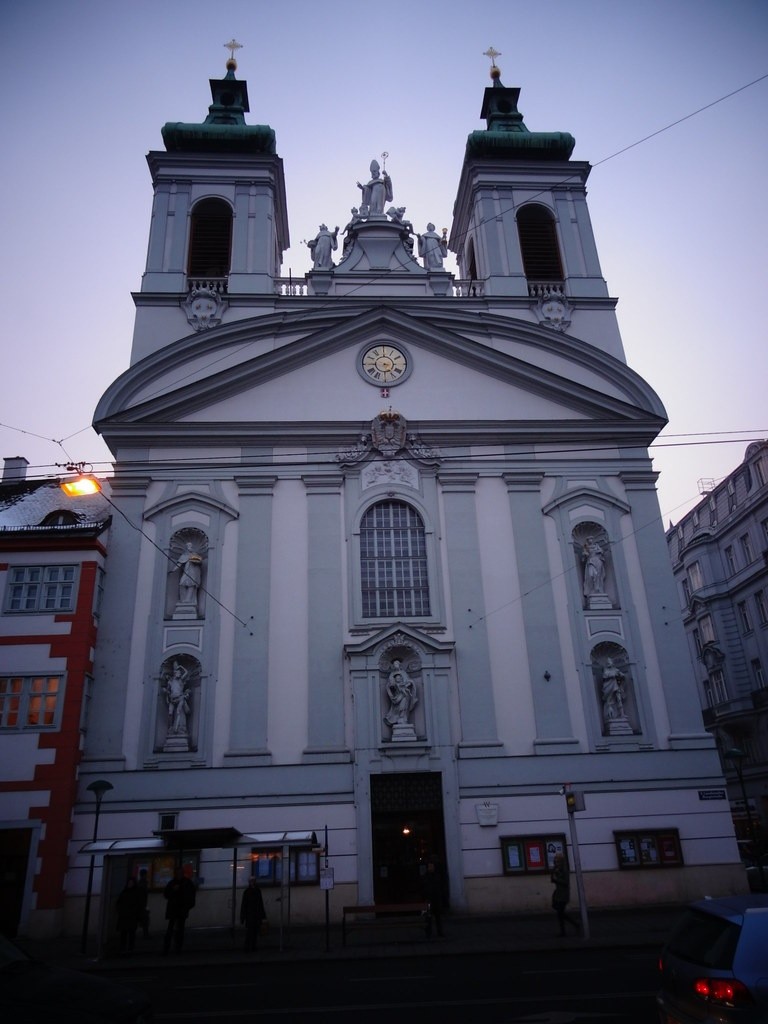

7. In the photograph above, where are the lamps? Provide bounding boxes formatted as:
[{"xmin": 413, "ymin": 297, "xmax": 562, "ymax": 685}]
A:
[{"xmin": 59, "ymin": 462, "xmax": 102, "ymax": 497}]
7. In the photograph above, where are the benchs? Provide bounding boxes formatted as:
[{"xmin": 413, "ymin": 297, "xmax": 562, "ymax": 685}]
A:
[{"xmin": 341, "ymin": 901, "xmax": 432, "ymax": 948}]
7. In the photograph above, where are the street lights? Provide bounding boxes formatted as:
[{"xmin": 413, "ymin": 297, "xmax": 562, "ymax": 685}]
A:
[{"xmin": 77, "ymin": 768, "xmax": 116, "ymax": 957}]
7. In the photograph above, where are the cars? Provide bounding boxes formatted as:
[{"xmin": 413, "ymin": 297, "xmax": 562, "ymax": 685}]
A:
[{"xmin": 648, "ymin": 890, "xmax": 768, "ymax": 1024}]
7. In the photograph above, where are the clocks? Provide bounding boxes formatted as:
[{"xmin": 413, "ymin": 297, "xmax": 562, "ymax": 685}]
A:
[{"xmin": 356, "ymin": 338, "xmax": 413, "ymax": 398}]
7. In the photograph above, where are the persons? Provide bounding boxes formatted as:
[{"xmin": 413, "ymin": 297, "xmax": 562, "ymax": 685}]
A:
[
  {"xmin": 162, "ymin": 866, "xmax": 196, "ymax": 955},
  {"xmin": 582, "ymin": 537, "xmax": 608, "ymax": 597},
  {"xmin": 341, "ymin": 160, "xmax": 406, "ymax": 234},
  {"xmin": 423, "ymin": 856, "xmax": 451, "ymax": 939},
  {"xmin": 309, "ymin": 224, "xmax": 341, "ymax": 272},
  {"xmin": 169, "ymin": 544, "xmax": 202, "ymax": 612},
  {"xmin": 601, "ymin": 658, "xmax": 629, "ymax": 717},
  {"xmin": 162, "ymin": 661, "xmax": 194, "ymax": 736},
  {"xmin": 116, "ymin": 868, "xmax": 151, "ymax": 959},
  {"xmin": 384, "ymin": 658, "xmax": 420, "ymax": 724},
  {"xmin": 239, "ymin": 877, "xmax": 268, "ymax": 948},
  {"xmin": 551, "ymin": 853, "xmax": 570, "ymax": 939},
  {"xmin": 416, "ymin": 223, "xmax": 449, "ymax": 268}
]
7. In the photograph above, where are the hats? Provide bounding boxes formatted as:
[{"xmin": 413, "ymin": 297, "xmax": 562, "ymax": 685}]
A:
[{"xmin": 248, "ymin": 875, "xmax": 257, "ymax": 880}]
